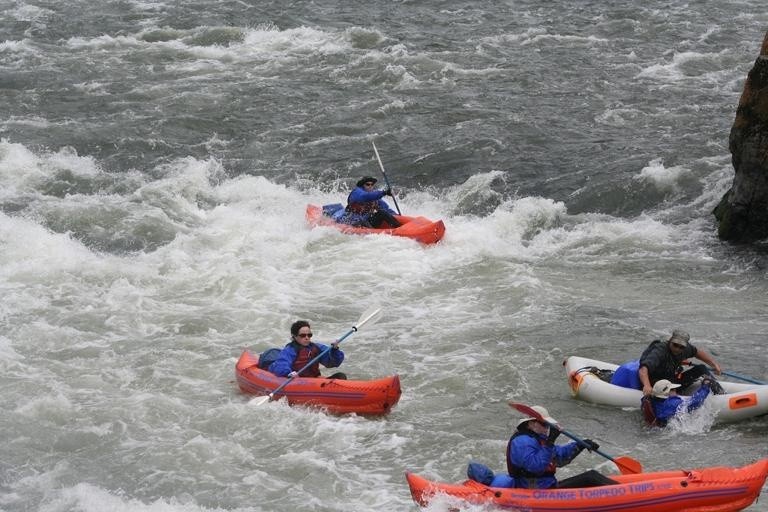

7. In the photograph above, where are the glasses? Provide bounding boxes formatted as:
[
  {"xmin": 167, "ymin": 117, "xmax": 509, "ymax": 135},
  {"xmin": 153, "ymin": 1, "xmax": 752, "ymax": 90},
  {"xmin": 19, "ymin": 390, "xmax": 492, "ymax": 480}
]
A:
[
  {"xmin": 365, "ymin": 183, "xmax": 373, "ymax": 186},
  {"xmin": 673, "ymin": 343, "xmax": 685, "ymax": 350},
  {"xmin": 297, "ymin": 334, "xmax": 312, "ymax": 337}
]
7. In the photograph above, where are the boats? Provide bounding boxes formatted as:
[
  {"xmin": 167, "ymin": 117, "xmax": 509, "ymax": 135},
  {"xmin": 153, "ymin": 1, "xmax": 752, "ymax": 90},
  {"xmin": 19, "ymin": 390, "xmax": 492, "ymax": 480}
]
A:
[
  {"xmin": 235, "ymin": 348, "xmax": 402, "ymax": 416},
  {"xmin": 563, "ymin": 356, "xmax": 767, "ymax": 424},
  {"xmin": 302, "ymin": 200, "xmax": 444, "ymax": 245},
  {"xmin": 397, "ymin": 458, "xmax": 767, "ymax": 512}
]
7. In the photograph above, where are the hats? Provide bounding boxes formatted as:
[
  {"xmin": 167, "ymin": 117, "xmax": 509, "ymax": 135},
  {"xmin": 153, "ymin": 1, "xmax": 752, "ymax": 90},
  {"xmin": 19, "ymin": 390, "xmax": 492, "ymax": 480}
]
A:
[
  {"xmin": 652, "ymin": 380, "xmax": 681, "ymax": 395},
  {"xmin": 356, "ymin": 176, "xmax": 377, "ymax": 187},
  {"xmin": 671, "ymin": 330, "xmax": 690, "ymax": 347},
  {"xmin": 517, "ymin": 406, "xmax": 558, "ymax": 424}
]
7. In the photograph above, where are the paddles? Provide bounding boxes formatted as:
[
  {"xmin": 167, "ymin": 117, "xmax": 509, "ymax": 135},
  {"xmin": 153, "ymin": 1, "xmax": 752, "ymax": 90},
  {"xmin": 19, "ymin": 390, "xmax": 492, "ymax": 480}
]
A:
[
  {"xmin": 372, "ymin": 142, "xmax": 401, "ymax": 215},
  {"xmin": 247, "ymin": 303, "xmax": 384, "ymax": 409},
  {"xmin": 681, "ymin": 359, "xmax": 768, "ymax": 385},
  {"xmin": 508, "ymin": 403, "xmax": 641, "ymax": 475}
]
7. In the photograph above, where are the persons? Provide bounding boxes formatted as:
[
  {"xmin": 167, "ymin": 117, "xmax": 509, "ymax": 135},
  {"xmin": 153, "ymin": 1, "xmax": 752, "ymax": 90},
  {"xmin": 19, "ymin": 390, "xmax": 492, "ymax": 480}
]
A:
[
  {"xmin": 643, "ymin": 375, "xmax": 717, "ymax": 430},
  {"xmin": 268, "ymin": 321, "xmax": 348, "ymax": 380},
  {"xmin": 638, "ymin": 329, "xmax": 726, "ymax": 397},
  {"xmin": 505, "ymin": 403, "xmax": 621, "ymax": 487},
  {"xmin": 337, "ymin": 175, "xmax": 401, "ymax": 228}
]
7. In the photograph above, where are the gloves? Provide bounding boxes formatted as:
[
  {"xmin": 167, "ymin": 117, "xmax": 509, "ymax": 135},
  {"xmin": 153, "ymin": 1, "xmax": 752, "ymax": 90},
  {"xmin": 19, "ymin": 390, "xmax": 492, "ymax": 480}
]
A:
[
  {"xmin": 331, "ymin": 339, "xmax": 339, "ymax": 348},
  {"xmin": 548, "ymin": 426, "xmax": 560, "ymax": 442},
  {"xmin": 288, "ymin": 371, "xmax": 299, "ymax": 378},
  {"xmin": 576, "ymin": 439, "xmax": 599, "ymax": 452},
  {"xmin": 386, "ymin": 189, "xmax": 392, "ymax": 196}
]
